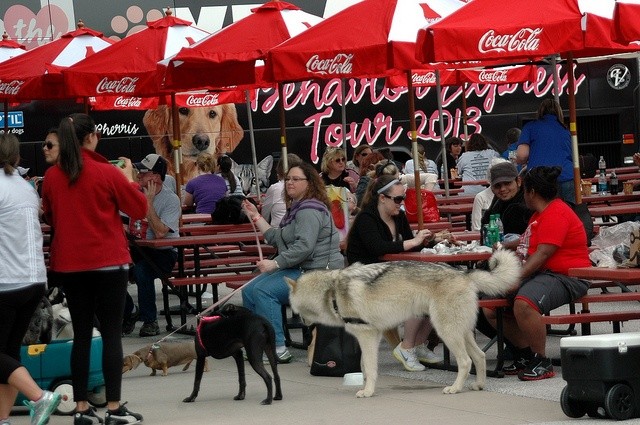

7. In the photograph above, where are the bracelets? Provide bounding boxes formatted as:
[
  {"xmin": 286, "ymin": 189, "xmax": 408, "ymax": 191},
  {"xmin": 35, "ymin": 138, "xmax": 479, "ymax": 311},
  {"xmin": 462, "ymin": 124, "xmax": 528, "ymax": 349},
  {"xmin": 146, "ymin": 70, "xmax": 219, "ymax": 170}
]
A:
[{"xmin": 252, "ymin": 214, "xmax": 263, "ymax": 222}]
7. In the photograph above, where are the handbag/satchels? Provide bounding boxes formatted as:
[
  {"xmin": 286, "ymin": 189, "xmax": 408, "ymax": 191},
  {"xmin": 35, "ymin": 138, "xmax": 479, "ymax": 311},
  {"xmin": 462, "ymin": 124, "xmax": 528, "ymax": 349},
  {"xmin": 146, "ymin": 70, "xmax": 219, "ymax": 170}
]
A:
[
  {"xmin": 404, "ymin": 188, "xmax": 440, "ymax": 223},
  {"xmin": 205, "ymin": 195, "xmax": 258, "ymax": 225},
  {"xmin": 326, "ymin": 184, "xmax": 351, "ymax": 242},
  {"xmin": 309, "ymin": 323, "xmax": 360, "ymax": 377}
]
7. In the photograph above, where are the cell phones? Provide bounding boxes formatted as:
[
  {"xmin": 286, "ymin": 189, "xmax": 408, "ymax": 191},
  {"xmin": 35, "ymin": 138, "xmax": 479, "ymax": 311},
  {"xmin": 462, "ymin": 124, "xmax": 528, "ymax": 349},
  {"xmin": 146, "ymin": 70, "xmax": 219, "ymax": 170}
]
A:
[{"xmin": 108, "ymin": 160, "xmax": 123, "ymax": 166}]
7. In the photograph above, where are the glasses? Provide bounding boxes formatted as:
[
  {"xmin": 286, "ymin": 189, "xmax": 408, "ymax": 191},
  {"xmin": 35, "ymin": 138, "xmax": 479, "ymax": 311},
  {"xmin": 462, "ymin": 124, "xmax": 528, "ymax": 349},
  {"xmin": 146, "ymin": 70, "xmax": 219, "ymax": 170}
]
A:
[
  {"xmin": 41, "ymin": 140, "xmax": 59, "ymax": 148},
  {"xmin": 285, "ymin": 175, "xmax": 309, "ymax": 181},
  {"xmin": 382, "ymin": 192, "xmax": 407, "ymax": 204},
  {"xmin": 361, "ymin": 153, "xmax": 369, "ymax": 156},
  {"xmin": 331, "ymin": 157, "xmax": 346, "ymax": 163}
]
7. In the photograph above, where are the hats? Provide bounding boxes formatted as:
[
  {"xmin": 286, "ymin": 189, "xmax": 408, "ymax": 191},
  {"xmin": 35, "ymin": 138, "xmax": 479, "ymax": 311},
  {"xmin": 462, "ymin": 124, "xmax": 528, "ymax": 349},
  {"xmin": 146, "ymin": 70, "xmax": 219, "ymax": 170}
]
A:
[
  {"xmin": 17, "ymin": 165, "xmax": 30, "ymax": 174},
  {"xmin": 133, "ymin": 153, "xmax": 168, "ymax": 177},
  {"xmin": 490, "ymin": 163, "xmax": 518, "ymax": 186}
]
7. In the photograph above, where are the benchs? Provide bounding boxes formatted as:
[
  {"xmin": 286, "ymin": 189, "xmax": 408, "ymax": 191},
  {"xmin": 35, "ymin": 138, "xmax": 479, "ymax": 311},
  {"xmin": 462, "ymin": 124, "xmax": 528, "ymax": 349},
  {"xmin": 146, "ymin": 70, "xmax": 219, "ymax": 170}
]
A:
[
  {"xmin": 173, "ymin": 256, "xmax": 268, "ymax": 269},
  {"xmin": 409, "ymin": 222, "xmax": 453, "ymax": 230},
  {"xmin": 226, "ymin": 280, "xmax": 251, "ymax": 288},
  {"xmin": 540, "ymin": 311, "xmax": 640, "ymax": 335},
  {"xmin": 478, "ymin": 292, "xmax": 639, "ymax": 379},
  {"xmin": 441, "ymin": 215, "xmax": 466, "ymax": 222},
  {"xmin": 172, "ymin": 272, "xmax": 264, "ymax": 335},
  {"xmin": 184, "ymin": 245, "xmax": 240, "ymax": 254}
]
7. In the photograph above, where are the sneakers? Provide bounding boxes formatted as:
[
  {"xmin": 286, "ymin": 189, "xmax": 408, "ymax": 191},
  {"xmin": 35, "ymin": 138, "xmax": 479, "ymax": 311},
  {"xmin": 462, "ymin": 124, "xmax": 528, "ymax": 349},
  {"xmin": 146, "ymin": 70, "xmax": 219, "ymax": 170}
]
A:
[
  {"xmin": 0, "ymin": 419, "xmax": 11, "ymax": 425},
  {"xmin": 518, "ymin": 353, "xmax": 556, "ymax": 381},
  {"xmin": 106, "ymin": 400, "xmax": 144, "ymax": 425},
  {"xmin": 23, "ymin": 390, "xmax": 60, "ymax": 425},
  {"xmin": 122, "ymin": 305, "xmax": 139, "ymax": 337},
  {"xmin": 415, "ymin": 342, "xmax": 445, "ymax": 363},
  {"xmin": 392, "ymin": 340, "xmax": 426, "ymax": 372},
  {"xmin": 139, "ymin": 317, "xmax": 160, "ymax": 336},
  {"xmin": 74, "ymin": 407, "xmax": 104, "ymax": 425},
  {"xmin": 499, "ymin": 355, "xmax": 535, "ymax": 375},
  {"xmin": 262, "ymin": 346, "xmax": 292, "ymax": 362}
]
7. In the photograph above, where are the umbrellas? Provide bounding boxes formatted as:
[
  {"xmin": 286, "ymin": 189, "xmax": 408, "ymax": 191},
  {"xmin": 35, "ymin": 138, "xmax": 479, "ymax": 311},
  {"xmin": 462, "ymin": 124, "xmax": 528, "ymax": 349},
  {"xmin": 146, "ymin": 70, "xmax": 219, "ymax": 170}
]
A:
[
  {"xmin": 0, "ymin": 31, "xmax": 28, "ymax": 134},
  {"xmin": 385, "ymin": 63, "xmax": 537, "ymax": 152},
  {"xmin": 415, "ymin": 0, "xmax": 640, "ymax": 206},
  {"xmin": 155, "ymin": 0, "xmax": 327, "ymax": 330},
  {"xmin": 16, "ymin": 5, "xmax": 257, "ymax": 316},
  {"xmin": 0, "ymin": 18, "xmax": 119, "ymax": 114},
  {"xmin": 262, "ymin": 0, "xmax": 538, "ymax": 234}
]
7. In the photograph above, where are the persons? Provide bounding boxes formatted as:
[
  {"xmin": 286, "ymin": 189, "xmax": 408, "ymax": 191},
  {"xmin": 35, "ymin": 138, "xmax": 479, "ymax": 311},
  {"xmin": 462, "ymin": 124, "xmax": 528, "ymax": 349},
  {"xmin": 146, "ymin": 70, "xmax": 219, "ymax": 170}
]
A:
[
  {"xmin": 240, "ymin": 162, "xmax": 344, "ymax": 366},
  {"xmin": 366, "ymin": 158, "xmax": 438, "ymax": 192},
  {"xmin": 216, "ymin": 156, "xmax": 246, "ymax": 196},
  {"xmin": 355, "ymin": 152, "xmax": 386, "ymax": 209},
  {"xmin": 502, "ymin": 128, "xmax": 523, "ymax": 174},
  {"xmin": 512, "ymin": 99, "xmax": 576, "ymax": 212},
  {"xmin": 346, "ymin": 175, "xmax": 445, "ymax": 372},
  {"xmin": 502, "ymin": 166, "xmax": 592, "ymax": 381},
  {"xmin": 42, "ymin": 128, "xmax": 60, "ymax": 165},
  {"xmin": 0, "ymin": 134, "xmax": 62, "ymax": 425},
  {"xmin": 471, "ymin": 158, "xmax": 512, "ymax": 232},
  {"xmin": 21, "ymin": 173, "xmax": 45, "ymax": 198},
  {"xmin": 41, "ymin": 113, "xmax": 148, "ymax": 425},
  {"xmin": 121, "ymin": 154, "xmax": 181, "ymax": 337},
  {"xmin": 633, "ymin": 152, "xmax": 640, "ymax": 166},
  {"xmin": 402, "ymin": 143, "xmax": 443, "ymax": 199},
  {"xmin": 346, "ymin": 143, "xmax": 374, "ymax": 177},
  {"xmin": 183, "ymin": 152, "xmax": 229, "ymax": 247},
  {"xmin": 435, "ymin": 137, "xmax": 463, "ymax": 190},
  {"xmin": 474, "ymin": 161, "xmax": 538, "ymax": 361},
  {"xmin": 318, "ymin": 146, "xmax": 360, "ymax": 194},
  {"xmin": 455, "ymin": 133, "xmax": 500, "ymax": 196},
  {"xmin": 261, "ymin": 153, "xmax": 303, "ymax": 231}
]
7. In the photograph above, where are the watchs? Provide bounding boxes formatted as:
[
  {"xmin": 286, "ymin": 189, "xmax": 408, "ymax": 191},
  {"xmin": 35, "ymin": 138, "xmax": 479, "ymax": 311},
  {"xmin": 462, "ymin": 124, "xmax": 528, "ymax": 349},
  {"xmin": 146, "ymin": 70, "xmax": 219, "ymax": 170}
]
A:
[{"xmin": 274, "ymin": 260, "xmax": 281, "ymax": 270}]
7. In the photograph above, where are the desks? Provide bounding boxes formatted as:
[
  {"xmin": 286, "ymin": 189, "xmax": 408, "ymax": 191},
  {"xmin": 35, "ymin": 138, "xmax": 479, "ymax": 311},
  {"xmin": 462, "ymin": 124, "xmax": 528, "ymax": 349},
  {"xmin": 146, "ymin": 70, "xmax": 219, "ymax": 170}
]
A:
[
  {"xmin": 436, "ymin": 195, "xmax": 475, "ymax": 204},
  {"xmin": 454, "ymin": 180, "xmax": 490, "ymax": 186},
  {"xmin": 182, "ymin": 213, "xmax": 212, "ymax": 222},
  {"xmin": 582, "ymin": 191, "xmax": 640, "ymax": 202},
  {"xmin": 433, "ymin": 189, "xmax": 465, "ymax": 195},
  {"xmin": 587, "ymin": 202, "xmax": 640, "ymax": 217},
  {"xmin": 568, "ymin": 267, "xmax": 640, "ymax": 280},
  {"xmin": 179, "ymin": 224, "xmax": 260, "ymax": 235},
  {"xmin": 454, "ymin": 235, "xmax": 482, "ymax": 240},
  {"xmin": 384, "ymin": 252, "xmax": 493, "ymax": 375},
  {"xmin": 437, "ymin": 179, "xmax": 458, "ymax": 183},
  {"xmin": 438, "ymin": 203, "xmax": 474, "ymax": 213},
  {"xmin": 581, "ymin": 173, "xmax": 640, "ymax": 183},
  {"xmin": 596, "ymin": 166, "xmax": 639, "ymax": 172},
  {"xmin": 135, "ymin": 232, "xmax": 265, "ymax": 334}
]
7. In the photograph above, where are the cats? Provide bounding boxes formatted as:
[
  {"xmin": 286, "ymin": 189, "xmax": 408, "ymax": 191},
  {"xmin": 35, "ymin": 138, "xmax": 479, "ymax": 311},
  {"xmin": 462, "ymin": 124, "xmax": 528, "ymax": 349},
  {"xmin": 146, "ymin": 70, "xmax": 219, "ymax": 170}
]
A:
[{"xmin": 222, "ymin": 154, "xmax": 274, "ymax": 199}]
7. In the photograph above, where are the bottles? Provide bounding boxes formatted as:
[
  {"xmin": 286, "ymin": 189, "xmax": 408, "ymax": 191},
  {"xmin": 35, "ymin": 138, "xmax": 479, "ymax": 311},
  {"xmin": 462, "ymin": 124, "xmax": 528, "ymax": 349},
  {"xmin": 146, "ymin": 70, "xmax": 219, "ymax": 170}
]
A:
[
  {"xmin": 609, "ymin": 172, "xmax": 618, "ymax": 195},
  {"xmin": 598, "ymin": 170, "xmax": 608, "ymax": 196},
  {"xmin": 487, "ymin": 214, "xmax": 498, "ymax": 246},
  {"xmin": 495, "ymin": 214, "xmax": 504, "ymax": 242},
  {"xmin": 599, "ymin": 156, "xmax": 606, "ymax": 176},
  {"xmin": 594, "ymin": 170, "xmax": 601, "ymax": 192},
  {"xmin": 481, "ymin": 224, "xmax": 489, "ymax": 245}
]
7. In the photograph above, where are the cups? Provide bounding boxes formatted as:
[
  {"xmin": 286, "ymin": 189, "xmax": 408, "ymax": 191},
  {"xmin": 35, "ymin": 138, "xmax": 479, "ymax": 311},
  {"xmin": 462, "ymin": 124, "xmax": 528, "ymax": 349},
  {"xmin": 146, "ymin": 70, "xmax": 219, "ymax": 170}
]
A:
[
  {"xmin": 582, "ymin": 183, "xmax": 592, "ymax": 197},
  {"xmin": 622, "ymin": 182, "xmax": 633, "ymax": 194}
]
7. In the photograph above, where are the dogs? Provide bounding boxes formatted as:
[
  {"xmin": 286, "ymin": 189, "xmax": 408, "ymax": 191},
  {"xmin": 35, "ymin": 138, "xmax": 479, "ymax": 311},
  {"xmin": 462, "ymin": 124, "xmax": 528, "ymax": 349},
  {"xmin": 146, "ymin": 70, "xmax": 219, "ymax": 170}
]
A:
[
  {"xmin": 284, "ymin": 248, "xmax": 521, "ymax": 398},
  {"xmin": 182, "ymin": 303, "xmax": 282, "ymax": 405},
  {"xmin": 143, "ymin": 101, "xmax": 244, "ymax": 186},
  {"xmin": 122, "ymin": 339, "xmax": 209, "ymax": 376}
]
